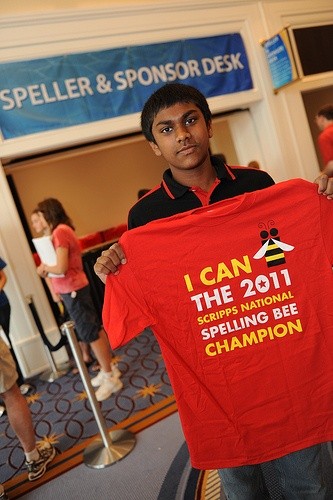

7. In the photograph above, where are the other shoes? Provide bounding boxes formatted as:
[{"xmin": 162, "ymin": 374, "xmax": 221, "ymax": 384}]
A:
[{"xmin": 20, "ymin": 384, "xmax": 32, "ymax": 395}]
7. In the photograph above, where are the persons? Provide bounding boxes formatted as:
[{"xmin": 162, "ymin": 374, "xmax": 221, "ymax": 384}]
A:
[
  {"xmin": 0, "ymin": 258, "xmax": 31, "ymax": 418},
  {"xmin": 94, "ymin": 83, "xmax": 333, "ymax": 500},
  {"xmin": 0, "ymin": 326, "xmax": 56, "ymax": 481},
  {"xmin": 31, "ymin": 209, "xmax": 116, "ymax": 375},
  {"xmin": 317, "ymin": 105, "xmax": 333, "ymax": 171},
  {"xmin": 248, "ymin": 160, "xmax": 260, "ymax": 169},
  {"xmin": 37, "ymin": 197, "xmax": 123, "ymax": 401}
]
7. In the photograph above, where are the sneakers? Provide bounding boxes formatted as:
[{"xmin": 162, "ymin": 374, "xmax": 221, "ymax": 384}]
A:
[
  {"xmin": 95, "ymin": 376, "xmax": 123, "ymax": 401},
  {"xmin": 25, "ymin": 440, "xmax": 56, "ymax": 480},
  {"xmin": 91, "ymin": 366, "xmax": 121, "ymax": 386}
]
